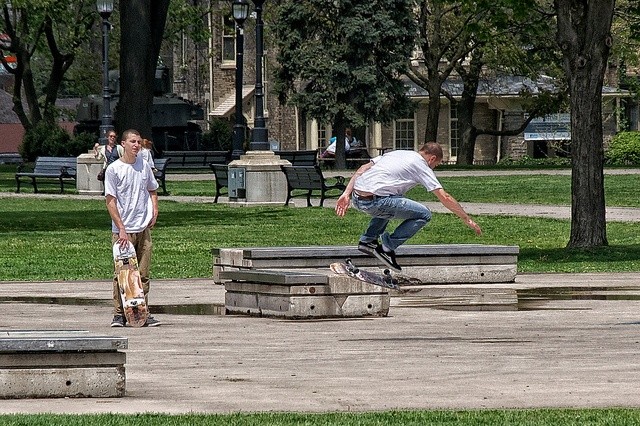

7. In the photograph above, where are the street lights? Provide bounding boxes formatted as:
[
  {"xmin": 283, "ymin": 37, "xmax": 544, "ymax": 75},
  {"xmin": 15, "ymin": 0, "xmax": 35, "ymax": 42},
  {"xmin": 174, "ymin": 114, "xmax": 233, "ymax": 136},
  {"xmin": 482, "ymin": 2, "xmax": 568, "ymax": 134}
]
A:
[
  {"xmin": 231, "ymin": 0, "xmax": 249, "ymax": 160},
  {"xmin": 95, "ymin": 0, "xmax": 116, "ymax": 144}
]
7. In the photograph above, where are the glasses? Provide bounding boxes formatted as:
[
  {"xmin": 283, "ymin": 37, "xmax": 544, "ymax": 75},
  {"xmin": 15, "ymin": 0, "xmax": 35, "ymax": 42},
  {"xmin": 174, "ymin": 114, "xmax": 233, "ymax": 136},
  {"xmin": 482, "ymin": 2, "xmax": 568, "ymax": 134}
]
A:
[{"xmin": 110, "ymin": 135, "xmax": 116, "ymax": 138}]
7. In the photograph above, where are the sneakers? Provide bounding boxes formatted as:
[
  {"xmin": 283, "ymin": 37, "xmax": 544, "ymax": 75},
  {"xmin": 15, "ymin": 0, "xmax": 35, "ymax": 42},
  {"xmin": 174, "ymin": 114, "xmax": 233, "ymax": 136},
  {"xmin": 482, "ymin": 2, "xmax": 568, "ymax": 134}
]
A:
[
  {"xmin": 143, "ymin": 317, "xmax": 162, "ymax": 326},
  {"xmin": 358, "ymin": 240, "xmax": 379, "ymax": 257},
  {"xmin": 372, "ymin": 245, "xmax": 401, "ymax": 273},
  {"xmin": 111, "ymin": 315, "xmax": 126, "ymax": 327}
]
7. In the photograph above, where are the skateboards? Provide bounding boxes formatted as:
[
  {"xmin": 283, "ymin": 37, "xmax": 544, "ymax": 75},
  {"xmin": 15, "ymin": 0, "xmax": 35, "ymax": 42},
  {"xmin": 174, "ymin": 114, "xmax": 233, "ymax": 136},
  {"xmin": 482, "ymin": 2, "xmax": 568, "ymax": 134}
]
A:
[
  {"xmin": 113, "ymin": 239, "xmax": 147, "ymax": 327},
  {"xmin": 330, "ymin": 258, "xmax": 399, "ymax": 292}
]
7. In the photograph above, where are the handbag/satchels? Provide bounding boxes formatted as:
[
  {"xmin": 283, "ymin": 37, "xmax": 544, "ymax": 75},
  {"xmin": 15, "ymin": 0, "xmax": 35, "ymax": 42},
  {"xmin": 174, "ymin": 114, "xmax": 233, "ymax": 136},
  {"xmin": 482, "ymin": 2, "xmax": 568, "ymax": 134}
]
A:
[{"xmin": 98, "ymin": 172, "xmax": 103, "ymax": 181}]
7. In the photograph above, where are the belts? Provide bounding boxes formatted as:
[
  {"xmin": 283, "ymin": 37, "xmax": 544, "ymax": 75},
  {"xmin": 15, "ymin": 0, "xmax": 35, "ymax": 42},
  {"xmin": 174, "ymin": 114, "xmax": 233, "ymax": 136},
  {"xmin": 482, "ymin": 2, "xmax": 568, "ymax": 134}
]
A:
[{"xmin": 358, "ymin": 195, "xmax": 381, "ymax": 200}]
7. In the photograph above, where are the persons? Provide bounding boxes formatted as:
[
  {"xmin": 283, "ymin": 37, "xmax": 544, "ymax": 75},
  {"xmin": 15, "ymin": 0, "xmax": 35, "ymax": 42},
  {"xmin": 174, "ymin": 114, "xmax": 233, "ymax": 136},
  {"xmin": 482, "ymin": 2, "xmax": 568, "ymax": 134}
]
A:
[
  {"xmin": 345, "ymin": 127, "xmax": 358, "ymax": 147},
  {"xmin": 334, "ymin": 141, "xmax": 481, "ymax": 273},
  {"xmin": 135, "ymin": 138, "xmax": 155, "ymax": 170},
  {"xmin": 104, "ymin": 128, "xmax": 164, "ymax": 327},
  {"xmin": 141, "ymin": 139, "xmax": 154, "ymax": 162},
  {"xmin": 94, "ymin": 130, "xmax": 124, "ymax": 196},
  {"xmin": 321, "ymin": 137, "xmax": 350, "ymax": 158}
]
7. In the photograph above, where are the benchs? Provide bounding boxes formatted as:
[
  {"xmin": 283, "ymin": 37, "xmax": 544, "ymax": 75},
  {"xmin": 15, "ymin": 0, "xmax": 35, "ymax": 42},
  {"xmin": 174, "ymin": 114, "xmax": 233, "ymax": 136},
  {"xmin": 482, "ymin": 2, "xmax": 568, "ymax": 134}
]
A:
[
  {"xmin": 212, "ymin": 243, "xmax": 520, "ymax": 285},
  {"xmin": 150, "ymin": 159, "xmax": 172, "ymax": 195},
  {"xmin": 161, "ymin": 150, "xmax": 230, "ymax": 170},
  {"xmin": 15, "ymin": 156, "xmax": 77, "ymax": 195},
  {"xmin": 219, "ymin": 266, "xmax": 390, "ymax": 322},
  {"xmin": 209, "ymin": 162, "xmax": 228, "ymax": 203},
  {"xmin": 279, "ymin": 164, "xmax": 347, "ymax": 207},
  {"xmin": 321, "ymin": 149, "xmax": 374, "ymax": 170},
  {"xmin": 273, "ymin": 149, "xmax": 318, "ymax": 166},
  {"xmin": 0, "ymin": 327, "xmax": 128, "ymax": 398}
]
